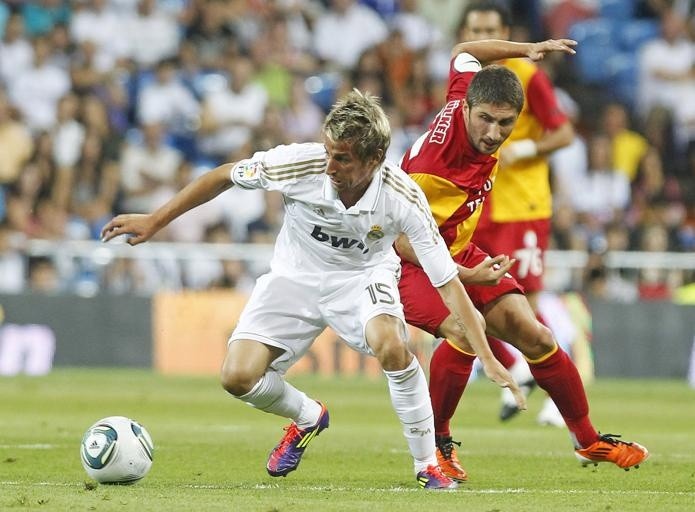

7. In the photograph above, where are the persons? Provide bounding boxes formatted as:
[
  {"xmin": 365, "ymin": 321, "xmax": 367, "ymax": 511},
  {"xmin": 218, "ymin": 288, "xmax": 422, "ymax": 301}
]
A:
[
  {"xmin": 512, "ymin": 288, "xmax": 597, "ymax": 388},
  {"xmin": 391, "ymin": 35, "xmax": 650, "ymax": 483},
  {"xmin": 451, "ymin": 5, "xmax": 583, "ymax": 428},
  {"xmin": 97, "ymin": 86, "xmax": 529, "ymax": 488},
  {"xmin": 0, "ymin": 0, "xmax": 695, "ymax": 301}
]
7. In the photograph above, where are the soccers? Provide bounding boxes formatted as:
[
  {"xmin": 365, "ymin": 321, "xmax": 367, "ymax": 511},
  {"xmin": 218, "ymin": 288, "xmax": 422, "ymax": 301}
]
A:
[{"xmin": 79, "ymin": 416, "xmax": 155, "ymax": 486}]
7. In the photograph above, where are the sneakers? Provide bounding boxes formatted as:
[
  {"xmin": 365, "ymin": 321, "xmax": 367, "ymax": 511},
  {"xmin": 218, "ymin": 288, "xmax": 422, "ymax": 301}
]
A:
[
  {"xmin": 266, "ymin": 399, "xmax": 329, "ymax": 476},
  {"xmin": 433, "ymin": 438, "xmax": 468, "ymax": 482},
  {"xmin": 416, "ymin": 467, "xmax": 456, "ymax": 491},
  {"xmin": 574, "ymin": 435, "xmax": 648, "ymax": 471},
  {"xmin": 502, "ymin": 379, "xmax": 534, "ymax": 418}
]
